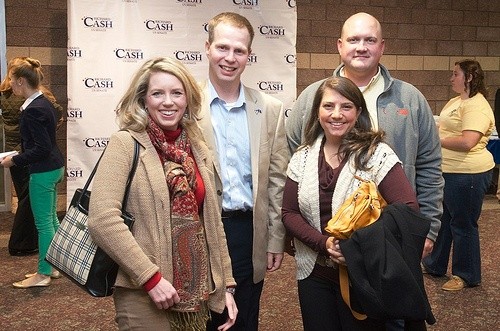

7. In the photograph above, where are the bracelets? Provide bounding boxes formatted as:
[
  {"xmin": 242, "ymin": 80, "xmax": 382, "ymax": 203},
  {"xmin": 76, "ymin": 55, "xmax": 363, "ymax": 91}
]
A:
[{"xmin": 226, "ymin": 287, "xmax": 235, "ymax": 295}]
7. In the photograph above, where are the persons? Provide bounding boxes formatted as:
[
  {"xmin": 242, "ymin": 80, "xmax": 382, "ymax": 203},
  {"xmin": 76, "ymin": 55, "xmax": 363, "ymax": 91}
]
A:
[
  {"xmin": 89, "ymin": 57, "xmax": 238, "ymax": 331},
  {"xmin": 200, "ymin": 12, "xmax": 288, "ymax": 331},
  {"xmin": 0, "ymin": 57, "xmax": 65, "ymax": 287},
  {"xmin": 422, "ymin": 59, "xmax": 495, "ymax": 291},
  {"xmin": 286, "ymin": 12, "xmax": 445, "ymax": 257},
  {"xmin": 282, "ymin": 77, "xmax": 420, "ymax": 331}
]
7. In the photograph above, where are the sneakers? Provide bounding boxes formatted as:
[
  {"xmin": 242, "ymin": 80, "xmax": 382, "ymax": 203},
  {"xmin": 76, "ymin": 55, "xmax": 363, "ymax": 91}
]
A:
[{"xmin": 441, "ymin": 275, "xmax": 468, "ymax": 291}]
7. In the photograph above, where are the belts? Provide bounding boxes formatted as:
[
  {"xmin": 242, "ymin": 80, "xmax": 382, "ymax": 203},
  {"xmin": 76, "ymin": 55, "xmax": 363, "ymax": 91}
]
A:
[{"xmin": 220, "ymin": 210, "xmax": 249, "ymax": 219}]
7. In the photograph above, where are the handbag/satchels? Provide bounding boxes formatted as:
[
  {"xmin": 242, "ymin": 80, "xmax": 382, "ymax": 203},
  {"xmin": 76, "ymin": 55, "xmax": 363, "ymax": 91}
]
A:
[
  {"xmin": 323, "ymin": 180, "xmax": 388, "ymax": 240},
  {"xmin": 45, "ymin": 129, "xmax": 140, "ymax": 298}
]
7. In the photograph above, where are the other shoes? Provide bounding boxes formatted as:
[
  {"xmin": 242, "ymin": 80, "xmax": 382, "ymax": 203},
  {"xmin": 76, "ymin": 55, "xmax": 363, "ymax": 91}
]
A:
[
  {"xmin": 12, "ymin": 275, "xmax": 51, "ymax": 288},
  {"xmin": 24, "ymin": 270, "xmax": 60, "ymax": 279}
]
